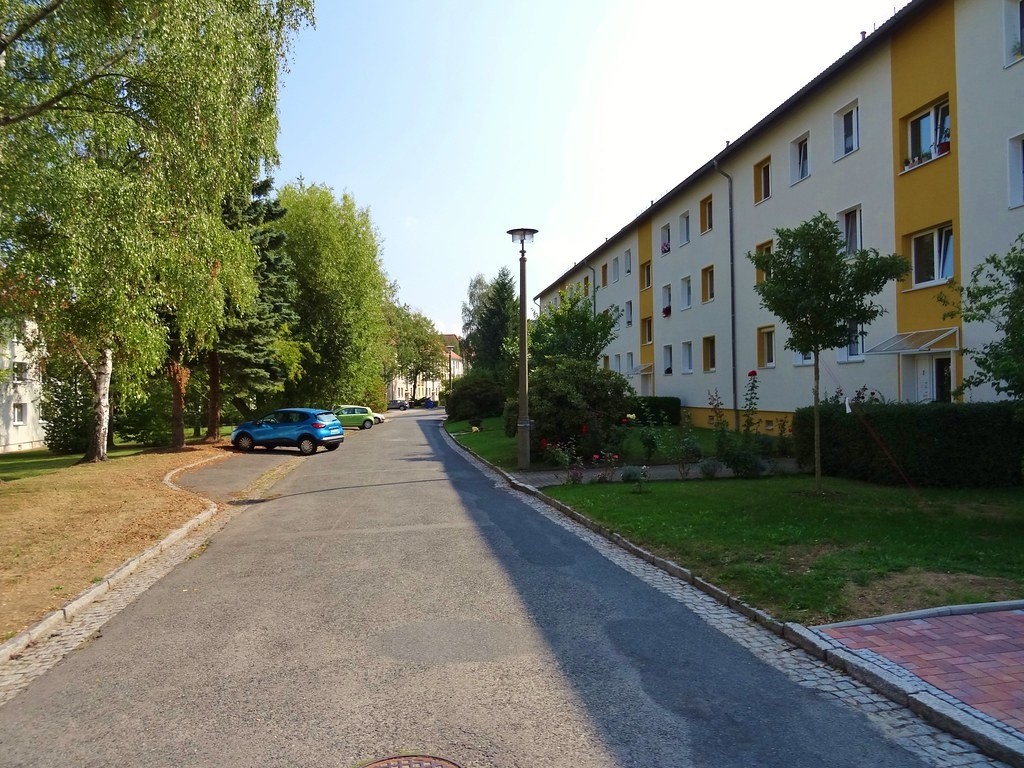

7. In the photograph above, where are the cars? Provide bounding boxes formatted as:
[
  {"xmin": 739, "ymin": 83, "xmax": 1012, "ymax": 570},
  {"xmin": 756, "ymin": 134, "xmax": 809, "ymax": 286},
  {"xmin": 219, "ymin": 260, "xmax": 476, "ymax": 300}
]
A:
[
  {"xmin": 387, "ymin": 400, "xmax": 410, "ymax": 411},
  {"xmin": 334, "ymin": 405, "xmax": 373, "ymax": 430},
  {"xmin": 372, "ymin": 413, "xmax": 385, "ymax": 425},
  {"xmin": 230, "ymin": 407, "xmax": 346, "ymax": 456}
]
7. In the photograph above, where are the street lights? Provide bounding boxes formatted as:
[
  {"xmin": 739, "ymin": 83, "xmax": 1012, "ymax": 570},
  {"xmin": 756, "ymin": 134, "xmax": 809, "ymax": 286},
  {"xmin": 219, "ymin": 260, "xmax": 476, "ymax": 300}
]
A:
[
  {"xmin": 506, "ymin": 227, "xmax": 539, "ymax": 471},
  {"xmin": 445, "ymin": 345, "xmax": 455, "ymax": 390}
]
